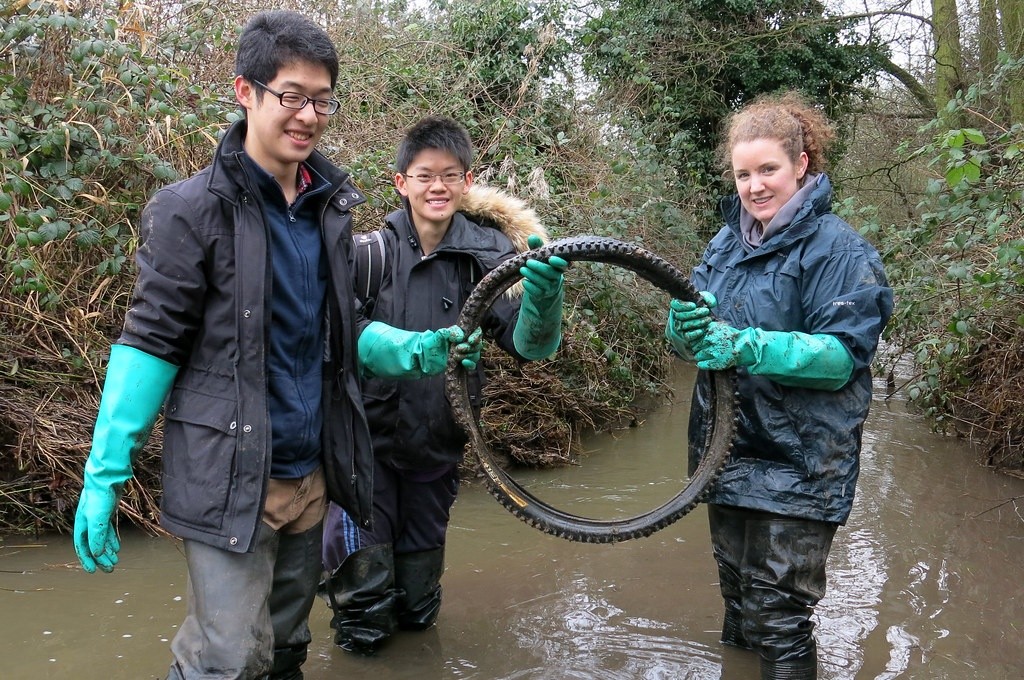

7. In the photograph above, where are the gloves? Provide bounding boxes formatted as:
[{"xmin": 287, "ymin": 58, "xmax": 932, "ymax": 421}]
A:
[
  {"xmin": 356, "ymin": 320, "xmax": 483, "ymax": 383},
  {"xmin": 73, "ymin": 343, "xmax": 180, "ymax": 573},
  {"xmin": 663, "ymin": 291, "xmax": 717, "ymax": 364},
  {"xmin": 512, "ymin": 234, "xmax": 569, "ymax": 360},
  {"xmin": 689, "ymin": 321, "xmax": 854, "ymax": 392}
]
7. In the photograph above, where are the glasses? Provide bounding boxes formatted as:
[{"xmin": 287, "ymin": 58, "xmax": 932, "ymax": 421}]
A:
[
  {"xmin": 401, "ymin": 171, "xmax": 465, "ymax": 184},
  {"xmin": 251, "ymin": 77, "xmax": 341, "ymax": 115}
]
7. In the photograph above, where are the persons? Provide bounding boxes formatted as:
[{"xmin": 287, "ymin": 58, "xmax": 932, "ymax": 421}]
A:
[
  {"xmin": 663, "ymin": 89, "xmax": 894, "ymax": 680},
  {"xmin": 75, "ymin": 11, "xmax": 484, "ymax": 680},
  {"xmin": 315, "ymin": 116, "xmax": 568, "ymax": 656}
]
